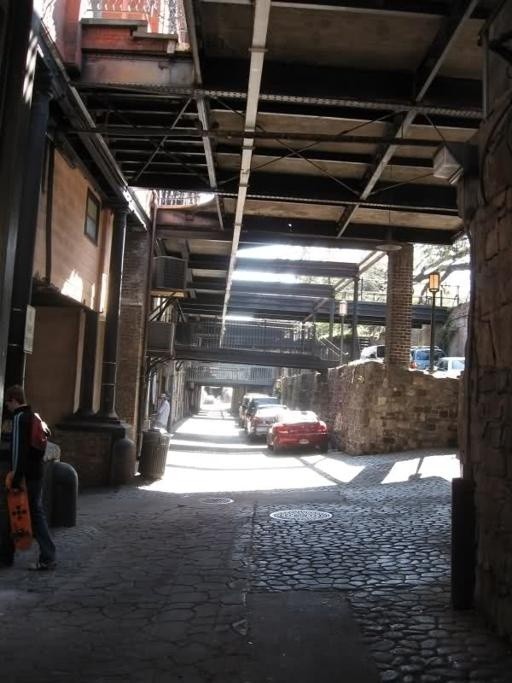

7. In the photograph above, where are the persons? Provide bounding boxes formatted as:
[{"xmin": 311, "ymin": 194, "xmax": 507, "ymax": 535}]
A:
[
  {"xmin": 0, "ymin": 385, "xmax": 62, "ymax": 571},
  {"xmin": 153, "ymin": 393, "xmax": 171, "ymax": 432}
]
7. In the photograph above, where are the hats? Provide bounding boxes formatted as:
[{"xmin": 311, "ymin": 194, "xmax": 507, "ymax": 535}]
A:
[{"xmin": 157, "ymin": 394, "xmax": 170, "ymax": 400}]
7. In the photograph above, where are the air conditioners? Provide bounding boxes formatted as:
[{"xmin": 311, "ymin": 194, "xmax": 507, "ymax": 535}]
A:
[
  {"xmin": 146, "ymin": 320, "xmax": 175, "ymax": 354},
  {"xmin": 432, "ymin": 146, "xmax": 469, "ymax": 180},
  {"xmin": 152, "ymin": 255, "xmax": 189, "ymax": 293}
]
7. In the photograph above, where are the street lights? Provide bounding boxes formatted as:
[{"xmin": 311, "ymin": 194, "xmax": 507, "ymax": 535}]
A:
[
  {"xmin": 338, "ymin": 300, "xmax": 347, "ymax": 366},
  {"xmin": 428, "ymin": 271, "xmax": 441, "ymax": 374}
]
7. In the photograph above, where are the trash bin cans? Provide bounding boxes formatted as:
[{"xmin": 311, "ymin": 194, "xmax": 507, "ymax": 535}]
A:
[{"xmin": 139, "ymin": 430, "xmax": 173, "ymax": 479}]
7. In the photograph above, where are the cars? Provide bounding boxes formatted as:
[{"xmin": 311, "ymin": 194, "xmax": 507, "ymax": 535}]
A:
[
  {"xmin": 266, "ymin": 410, "xmax": 329, "ymax": 453},
  {"xmin": 238, "ymin": 392, "xmax": 269, "ymax": 423},
  {"xmin": 244, "ymin": 403, "xmax": 289, "ymax": 440},
  {"xmin": 242, "ymin": 396, "xmax": 280, "ymax": 430},
  {"xmin": 409, "ymin": 345, "xmax": 466, "ymax": 379}
]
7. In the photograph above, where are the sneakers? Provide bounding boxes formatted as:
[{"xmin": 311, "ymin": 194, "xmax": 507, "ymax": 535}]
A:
[{"xmin": 28, "ymin": 558, "xmax": 51, "ymax": 570}]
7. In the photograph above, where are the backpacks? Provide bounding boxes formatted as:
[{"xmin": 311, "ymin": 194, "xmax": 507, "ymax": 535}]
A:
[{"xmin": 30, "ymin": 412, "xmax": 51, "ymax": 451}]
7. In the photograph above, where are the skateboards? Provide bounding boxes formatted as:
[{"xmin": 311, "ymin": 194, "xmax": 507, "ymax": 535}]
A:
[{"xmin": 4, "ymin": 472, "xmax": 32, "ymax": 552}]
